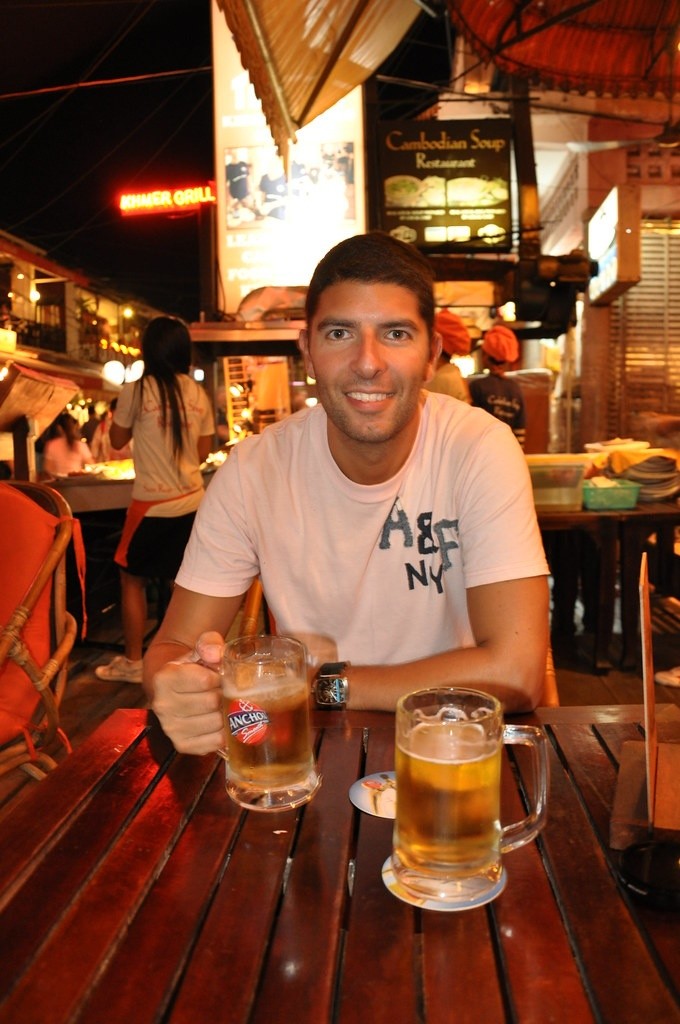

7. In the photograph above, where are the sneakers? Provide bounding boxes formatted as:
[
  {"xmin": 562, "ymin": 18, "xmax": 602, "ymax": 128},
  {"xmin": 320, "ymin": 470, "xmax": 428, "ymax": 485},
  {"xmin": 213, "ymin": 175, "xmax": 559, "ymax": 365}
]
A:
[{"xmin": 95, "ymin": 656, "xmax": 146, "ymax": 684}]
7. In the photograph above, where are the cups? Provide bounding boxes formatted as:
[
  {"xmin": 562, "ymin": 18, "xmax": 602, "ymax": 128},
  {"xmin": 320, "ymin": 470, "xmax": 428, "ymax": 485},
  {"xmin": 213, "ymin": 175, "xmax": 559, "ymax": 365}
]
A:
[
  {"xmin": 196, "ymin": 633, "xmax": 322, "ymax": 813},
  {"xmin": 392, "ymin": 686, "xmax": 548, "ymax": 901}
]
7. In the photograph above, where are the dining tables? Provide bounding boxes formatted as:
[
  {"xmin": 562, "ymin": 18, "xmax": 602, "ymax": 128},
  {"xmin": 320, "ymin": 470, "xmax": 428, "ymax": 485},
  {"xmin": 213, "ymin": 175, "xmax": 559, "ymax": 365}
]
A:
[{"xmin": 0, "ymin": 703, "xmax": 680, "ymax": 1024}]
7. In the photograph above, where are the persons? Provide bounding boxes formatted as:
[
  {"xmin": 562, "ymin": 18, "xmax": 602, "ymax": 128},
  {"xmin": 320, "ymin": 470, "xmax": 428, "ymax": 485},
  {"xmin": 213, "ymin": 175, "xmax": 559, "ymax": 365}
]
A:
[
  {"xmin": 467, "ymin": 325, "xmax": 527, "ymax": 452},
  {"xmin": 654, "ymin": 664, "xmax": 680, "ymax": 688},
  {"xmin": 0, "ymin": 460, "xmax": 11, "ymax": 480},
  {"xmin": 95, "ymin": 315, "xmax": 215, "ymax": 683},
  {"xmin": 225, "ymin": 142, "xmax": 355, "ymax": 221},
  {"xmin": 82, "ymin": 406, "xmax": 100, "ymax": 442},
  {"xmin": 141, "ymin": 232, "xmax": 551, "ymax": 757},
  {"xmin": 91, "ymin": 397, "xmax": 133, "ymax": 462},
  {"xmin": 424, "ymin": 310, "xmax": 472, "ymax": 406},
  {"xmin": 42, "ymin": 414, "xmax": 94, "ymax": 477},
  {"xmin": 545, "ymin": 381, "xmax": 600, "ymax": 667}
]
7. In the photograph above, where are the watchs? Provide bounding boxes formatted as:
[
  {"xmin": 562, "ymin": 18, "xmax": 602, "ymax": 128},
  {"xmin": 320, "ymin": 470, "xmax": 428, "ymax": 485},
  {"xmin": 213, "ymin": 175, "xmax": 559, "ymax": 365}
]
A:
[{"xmin": 310, "ymin": 662, "xmax": 351, "ymax": 710}]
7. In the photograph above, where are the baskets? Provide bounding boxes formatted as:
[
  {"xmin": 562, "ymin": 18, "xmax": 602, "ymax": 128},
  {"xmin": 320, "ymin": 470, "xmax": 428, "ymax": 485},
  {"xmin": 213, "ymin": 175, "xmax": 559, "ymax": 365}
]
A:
[{"xmin": 582, "ymin": 477, "xmax": 643, "ymax": 510}]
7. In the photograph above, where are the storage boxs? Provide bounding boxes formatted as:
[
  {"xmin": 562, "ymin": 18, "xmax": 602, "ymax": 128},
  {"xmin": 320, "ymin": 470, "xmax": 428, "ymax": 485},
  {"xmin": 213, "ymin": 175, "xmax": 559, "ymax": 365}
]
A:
[
  {"xmin": 582, "ymin": 473, "xmax": 642, "ymax": 509},
  {"xmin": 522, "ymin": 452, "xmax": 589, "ymax": 514}
]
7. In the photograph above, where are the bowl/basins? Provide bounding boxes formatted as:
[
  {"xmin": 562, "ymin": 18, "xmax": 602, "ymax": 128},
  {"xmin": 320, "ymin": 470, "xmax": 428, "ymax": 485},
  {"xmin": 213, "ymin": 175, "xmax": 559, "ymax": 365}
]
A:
[{"xmin": 618, "ymin": 840, "xmax": 679, "ymax": 911}]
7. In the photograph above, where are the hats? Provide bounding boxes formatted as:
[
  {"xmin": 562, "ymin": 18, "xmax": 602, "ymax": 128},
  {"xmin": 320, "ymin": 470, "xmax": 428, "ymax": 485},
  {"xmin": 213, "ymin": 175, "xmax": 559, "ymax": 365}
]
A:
[
  {"xmin": 433, "ymin": 308, "xmax": 471, "ymax": 357},
  {"xmin": 482, "ymin": 325, "xmax": 519, "ymax": 362}
]
